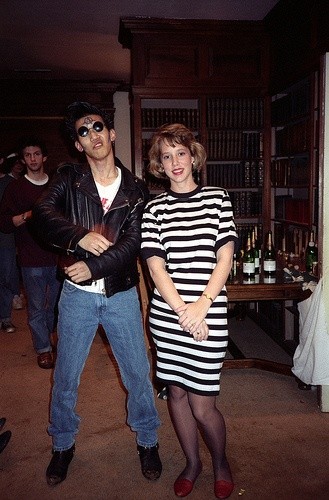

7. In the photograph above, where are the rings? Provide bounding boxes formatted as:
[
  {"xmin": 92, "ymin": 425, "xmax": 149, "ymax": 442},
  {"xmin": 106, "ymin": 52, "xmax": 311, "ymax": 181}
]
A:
[{"xmin": 197, "ymin": 332, "xmax": 201, "ymax": 334}]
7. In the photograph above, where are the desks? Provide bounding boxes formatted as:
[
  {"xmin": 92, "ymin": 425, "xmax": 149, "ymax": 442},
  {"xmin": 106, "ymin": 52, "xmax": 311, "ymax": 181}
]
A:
[{"xmin": 218, "ymin": 256, "xmax": 315, "ymax": 391}]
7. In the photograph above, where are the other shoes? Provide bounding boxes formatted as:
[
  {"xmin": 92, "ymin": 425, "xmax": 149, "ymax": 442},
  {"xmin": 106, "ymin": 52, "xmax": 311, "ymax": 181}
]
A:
[
  {"xmin": 214, "ymin": 479, "xmax": 234, "ymax": 498},
  {"xmin": 37, "ymin": 351, "xmax": 52, "ymax": 369},
  {"xmin": 174, "ymin": 461, "xmax": 202, "ymax": 497},
  {"xmin": 2, "ymin": 320, "xmax": 15, "ymax": 332},
  {"xmin": 0, "ymin": 430, "xmax": 11, "ymax": 452},
  {"xmin": 12, "ymin": 295, "xmax": 23, "ymax": 310},
  {"xmin": 0, "ymin": 417, "xmax": 6, "ymax": 428}
]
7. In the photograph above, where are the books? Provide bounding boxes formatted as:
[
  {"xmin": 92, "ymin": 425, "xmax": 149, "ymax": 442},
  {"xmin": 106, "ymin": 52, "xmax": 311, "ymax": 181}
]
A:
[
  {"xmin": 270, "ymin": 86, "xmax": 310, "ymax": 256},
  {"xmin": 142, "ymin": 97, "xmax": 264, "ymax": 258}
]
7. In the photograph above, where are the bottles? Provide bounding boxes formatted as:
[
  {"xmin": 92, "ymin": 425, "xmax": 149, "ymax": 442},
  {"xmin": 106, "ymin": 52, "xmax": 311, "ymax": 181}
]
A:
[
  {"xmin": 305, "ymin": 230, "xmax": 318, "ymax": 272},
  {"xmin": 225, "ymin": 250, "xmax": 237, "ymax": 285},
  {"xmin": 242, "ymin": 226, "xmax": 262, "ymax": 285},
  {"xmin": 262, "ymin": 230, "xmax": 277, "ymax": 284}
]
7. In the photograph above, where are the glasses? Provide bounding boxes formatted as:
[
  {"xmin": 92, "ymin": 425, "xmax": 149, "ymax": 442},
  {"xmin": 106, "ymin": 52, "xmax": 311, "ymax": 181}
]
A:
[{"xmin": 77, "ymin": 121, "xmax": 106, "ymax": 137}]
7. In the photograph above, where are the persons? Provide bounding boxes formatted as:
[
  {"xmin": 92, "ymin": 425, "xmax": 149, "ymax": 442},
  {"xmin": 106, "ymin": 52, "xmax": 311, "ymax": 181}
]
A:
[
  {"xmin": 38, "ymin": 111, "xmax": 163, "ymax": 486},
  {"xmin": 0, "ymin": 137, "xmax": 62, "ymax": 368},
  {"xmin": 141, "ymin": 125, "xmax": 238, "ymax": 499}
]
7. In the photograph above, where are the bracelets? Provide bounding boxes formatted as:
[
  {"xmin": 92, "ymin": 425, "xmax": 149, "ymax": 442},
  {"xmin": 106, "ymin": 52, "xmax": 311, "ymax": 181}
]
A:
[
  {"xmin": 202, "ymin": 293, "xmax": 213, "ymax": 303},
  {"xmin": 22, "ymin": 214, "xmax": 27, "ymax": 221}
]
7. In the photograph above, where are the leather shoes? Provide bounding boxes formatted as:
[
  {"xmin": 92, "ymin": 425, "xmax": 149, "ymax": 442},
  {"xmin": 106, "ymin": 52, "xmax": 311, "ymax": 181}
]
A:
[
  {"xmin": 136, "ymin": 441, "xmax": 162, "ymax": 480},
  {"xmin": 46, "ymin": 443, "xmax": 76, "ymax": 485}
]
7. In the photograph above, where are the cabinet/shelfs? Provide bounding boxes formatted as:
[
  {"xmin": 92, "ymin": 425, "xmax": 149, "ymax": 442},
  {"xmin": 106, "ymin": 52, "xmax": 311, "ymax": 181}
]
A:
[{"xmin": 119, "ymin": 0, "xmax": 319, "ymax": 263}]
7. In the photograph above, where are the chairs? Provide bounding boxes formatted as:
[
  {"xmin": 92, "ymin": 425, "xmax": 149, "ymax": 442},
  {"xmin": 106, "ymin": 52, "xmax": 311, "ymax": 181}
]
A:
[{"xmin": 137, "ymin": 259, "xmax": 155, "ymax": 350}]
